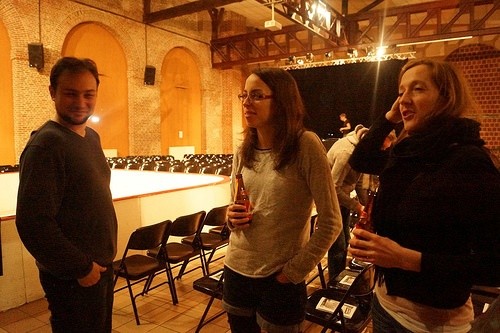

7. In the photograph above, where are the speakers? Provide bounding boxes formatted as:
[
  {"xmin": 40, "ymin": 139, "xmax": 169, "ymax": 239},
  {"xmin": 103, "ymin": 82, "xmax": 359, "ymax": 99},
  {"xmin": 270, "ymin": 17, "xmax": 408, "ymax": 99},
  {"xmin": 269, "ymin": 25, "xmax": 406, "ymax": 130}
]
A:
[
  {"xmin": 28, "ymin": 43, "xmax": 45, "ymax": 69},
  {"xmin": 144, "ymin": 65, "xmax": 156, "ymax": 86}
]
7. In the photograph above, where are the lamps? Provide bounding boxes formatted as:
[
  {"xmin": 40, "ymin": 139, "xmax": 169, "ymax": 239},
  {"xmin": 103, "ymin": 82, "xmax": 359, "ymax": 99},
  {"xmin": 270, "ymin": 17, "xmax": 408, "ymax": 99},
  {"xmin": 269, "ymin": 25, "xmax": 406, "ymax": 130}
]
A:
[{"xmin": 288, "ymin": 43, "xmax": 398, "ymax": 64}]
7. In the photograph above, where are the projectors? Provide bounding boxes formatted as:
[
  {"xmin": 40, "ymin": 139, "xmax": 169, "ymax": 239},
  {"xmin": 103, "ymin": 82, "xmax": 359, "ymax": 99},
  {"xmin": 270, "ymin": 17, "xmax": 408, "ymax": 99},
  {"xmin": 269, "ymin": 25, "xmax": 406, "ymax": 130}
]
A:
[{"xmin": 265, "ymin": 21, "xmax": 283, "ymax": 32}]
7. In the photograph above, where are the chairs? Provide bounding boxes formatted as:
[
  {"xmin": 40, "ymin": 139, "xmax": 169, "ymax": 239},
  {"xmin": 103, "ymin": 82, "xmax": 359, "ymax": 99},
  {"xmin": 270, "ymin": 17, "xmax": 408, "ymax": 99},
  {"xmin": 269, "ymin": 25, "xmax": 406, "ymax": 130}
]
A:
[
  {"xmin": 303, "ymin": 194, "xmax": 375, "ymax": 333},
  {"xmin": 105, "ymin": 154, "xmax": 234, "ymax": 176},
  {"xmin": 112, "ymin": 205, "xmax": 231, "ymax": 332}
]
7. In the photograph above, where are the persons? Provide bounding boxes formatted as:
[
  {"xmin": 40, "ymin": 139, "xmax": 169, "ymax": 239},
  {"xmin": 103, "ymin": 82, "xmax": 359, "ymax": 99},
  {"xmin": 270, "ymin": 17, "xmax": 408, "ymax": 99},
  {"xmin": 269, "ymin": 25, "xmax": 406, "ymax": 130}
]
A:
[
  {"xmin": 340, "ymin": 113, "xmax": 351, "ymax": 137},
  {"xmin": 348, "ymin": 57, "xmax": 500, "ymax": 333},
  {"xmin": 222, "ymin": 69, "xmax": 343, "ymax": 333},
  {"xmin": 16, "ymin": 56, "xmax": 118, "ymax": 333},
  {"xmin": 326, "ymin": 124, "xmax": 397, "ymax": 278}
]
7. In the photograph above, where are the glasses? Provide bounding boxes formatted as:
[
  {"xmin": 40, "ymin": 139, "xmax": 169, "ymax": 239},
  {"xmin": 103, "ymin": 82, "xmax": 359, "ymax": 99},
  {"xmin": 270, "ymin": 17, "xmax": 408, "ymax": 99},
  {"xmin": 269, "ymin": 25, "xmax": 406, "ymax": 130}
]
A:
[{"xmin": 238, "ymin": 92, "xmax": 272, "ymax": 102}]
